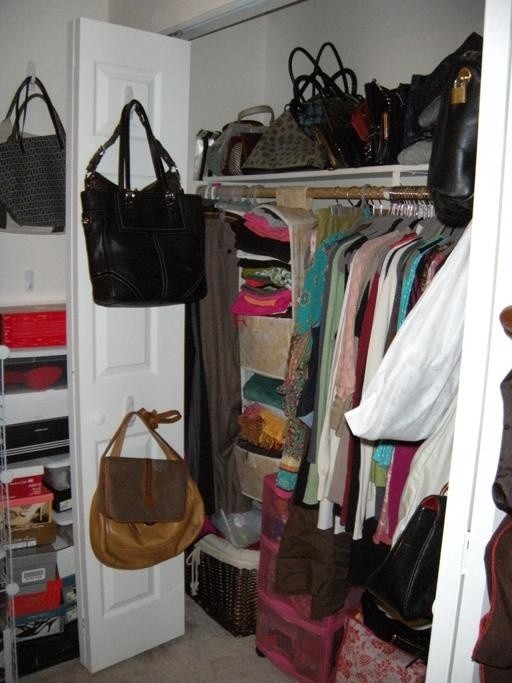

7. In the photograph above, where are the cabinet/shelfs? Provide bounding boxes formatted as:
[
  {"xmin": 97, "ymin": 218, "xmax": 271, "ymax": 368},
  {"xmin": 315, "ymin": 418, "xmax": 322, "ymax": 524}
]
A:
[{"xmin": 0, "ymin": 345, "xmax": 80, "ymax": 682}]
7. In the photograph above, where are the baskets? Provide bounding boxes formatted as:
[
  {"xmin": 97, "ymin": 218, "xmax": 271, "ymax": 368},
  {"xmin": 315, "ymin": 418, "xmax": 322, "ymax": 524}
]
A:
[{"xmin": 184, "ymin": 544, "xmax": 259, "ymax": 639}]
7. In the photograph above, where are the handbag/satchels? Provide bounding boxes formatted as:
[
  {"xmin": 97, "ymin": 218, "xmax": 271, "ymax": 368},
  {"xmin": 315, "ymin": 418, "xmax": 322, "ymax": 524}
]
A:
[
  {"xmin": 80, "ymin": 171, "xmax": 208, "ymax": 306},
  {"xmin": 361, "ymin": 482, "xmax": 447, "ymax": 664},
  {"xmin": 192, "ymin": 73, "xmax": 442, "ymax": 176},
  {"xmin": 425, "ymin": 56, "xmax": 478, "ymax": 227},
  {"xmin": 0, "ymin": 116, "xmax": 66, "ymax": 233},
  {"xmin": 100, "ymin": 411, "xmax": 190, "ymax": 523},
  {"xmin": 89, "ymin": 408, "xmax": 205, "ymax": 570}
]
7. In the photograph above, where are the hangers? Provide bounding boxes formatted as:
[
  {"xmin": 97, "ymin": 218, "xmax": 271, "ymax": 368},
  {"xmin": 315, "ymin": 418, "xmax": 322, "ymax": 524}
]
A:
[{"xmin": 199, "ymin": 182, "xmax": 436, "ymax": 221}]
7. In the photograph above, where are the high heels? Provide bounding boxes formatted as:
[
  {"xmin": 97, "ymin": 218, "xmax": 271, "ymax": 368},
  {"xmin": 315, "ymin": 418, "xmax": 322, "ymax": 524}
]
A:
[
  {"xmin": 6, "ymin": 502, "xmax": 47, "ymax": 526},
  {"xmin": 16, "ymin": 615, "xmax": 57, "ymax": 637}
]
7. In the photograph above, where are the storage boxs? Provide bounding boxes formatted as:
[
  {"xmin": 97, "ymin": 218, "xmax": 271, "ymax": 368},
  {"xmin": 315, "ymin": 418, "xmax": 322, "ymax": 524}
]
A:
[
  {"xmin": 253, "ymin": 471, "xmax": 367, "ymax": 681},
  {"xmin": 0, "ymin": 452, "xmax": 78, "ymax": 641}
]
